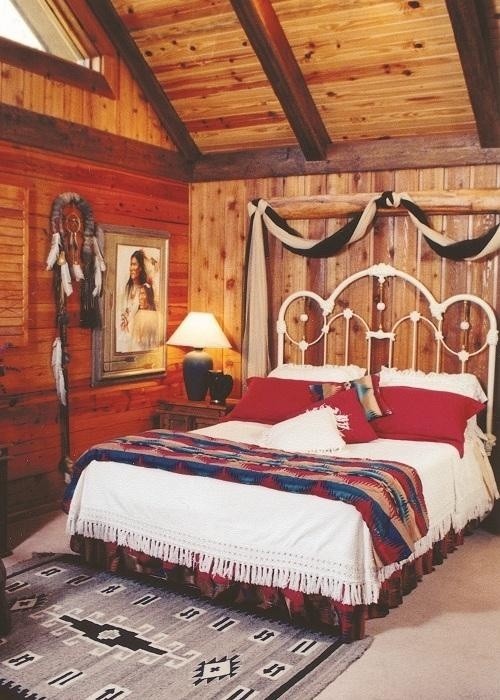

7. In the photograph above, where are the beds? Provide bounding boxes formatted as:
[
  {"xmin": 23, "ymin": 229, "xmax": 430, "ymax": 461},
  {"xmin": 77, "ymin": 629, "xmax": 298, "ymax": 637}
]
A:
[{"xmin": 64, "ymin": 260, "xmax": 500, "ymax": 645}]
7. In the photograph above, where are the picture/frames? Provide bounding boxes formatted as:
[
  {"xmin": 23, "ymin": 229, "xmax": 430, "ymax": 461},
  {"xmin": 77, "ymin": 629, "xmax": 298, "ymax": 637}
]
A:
[{"xmin": 91, "ymin": 225, "xmax": 171, "ymax": 388}]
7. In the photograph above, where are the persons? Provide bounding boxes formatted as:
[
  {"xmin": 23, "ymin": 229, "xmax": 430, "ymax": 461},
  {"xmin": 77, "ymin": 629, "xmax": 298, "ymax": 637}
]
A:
[
  {"xmin": 122, "ymin": 250, "xmax": 146, "ymax": 329},
  {"xmin": 134, "ymin": 280, "xmax": 156, "ymax": 346}
]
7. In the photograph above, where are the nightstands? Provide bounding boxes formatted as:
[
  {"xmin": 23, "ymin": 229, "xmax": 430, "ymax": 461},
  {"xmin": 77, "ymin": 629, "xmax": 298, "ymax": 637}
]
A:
[{"xmin": 157, "ymin": 398, "xmax": 239, "ymax": 430}]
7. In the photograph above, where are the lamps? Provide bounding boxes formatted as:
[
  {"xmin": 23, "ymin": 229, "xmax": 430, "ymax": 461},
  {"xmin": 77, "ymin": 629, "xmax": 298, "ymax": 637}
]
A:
[{"xmin": 167, "ymin": 311, "xmax": 232, "ymax": 401}]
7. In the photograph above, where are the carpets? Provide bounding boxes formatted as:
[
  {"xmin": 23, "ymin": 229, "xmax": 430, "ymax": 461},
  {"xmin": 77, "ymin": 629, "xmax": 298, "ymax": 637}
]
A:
[{"xmin": 0, "ymin": 544, "xmax": 376, "ymax": 699}]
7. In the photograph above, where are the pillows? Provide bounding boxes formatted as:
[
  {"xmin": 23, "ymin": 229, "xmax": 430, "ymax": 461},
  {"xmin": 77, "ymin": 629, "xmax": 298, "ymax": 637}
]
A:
[{"xmin": 219, "ymin": 359, "xmax": 488, "ymax": 455}]
